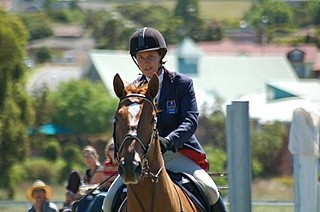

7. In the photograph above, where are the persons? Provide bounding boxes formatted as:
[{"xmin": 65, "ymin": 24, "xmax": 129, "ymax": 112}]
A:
[
  {"xmin": 25, "ymin": 180, "xmax": 59, "ymax": 212},
  {"xmin": 90, "ymin": 139, "xmax": 120, "ymax": 189},
  {"xmin": 101, "ymin": 27, "xmax": 226, "ymax": 212},
  {"xmin": 81, "ymin": 145, "xmax": 102, "ymax": 185}
]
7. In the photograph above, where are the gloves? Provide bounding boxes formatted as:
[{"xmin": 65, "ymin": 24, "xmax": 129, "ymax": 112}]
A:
[{"xmin": 158, "ymin": 137, "xmax": 169, "ymax": 155}]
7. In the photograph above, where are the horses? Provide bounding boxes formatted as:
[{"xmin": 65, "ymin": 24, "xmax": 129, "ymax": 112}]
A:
[{"xmin": 109, "ymin": 71, "xmax": 208, "ymax": 212}]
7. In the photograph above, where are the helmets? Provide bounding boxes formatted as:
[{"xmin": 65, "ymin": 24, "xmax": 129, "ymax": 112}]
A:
[{"xmin": 129, "ymin": 27, "xmax": 168, "ymax": 58}]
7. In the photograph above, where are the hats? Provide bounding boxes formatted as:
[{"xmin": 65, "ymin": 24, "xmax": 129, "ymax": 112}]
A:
[{"xmin": 25, "ymin": 180, "xmax": 51, "ymax": 205}]
